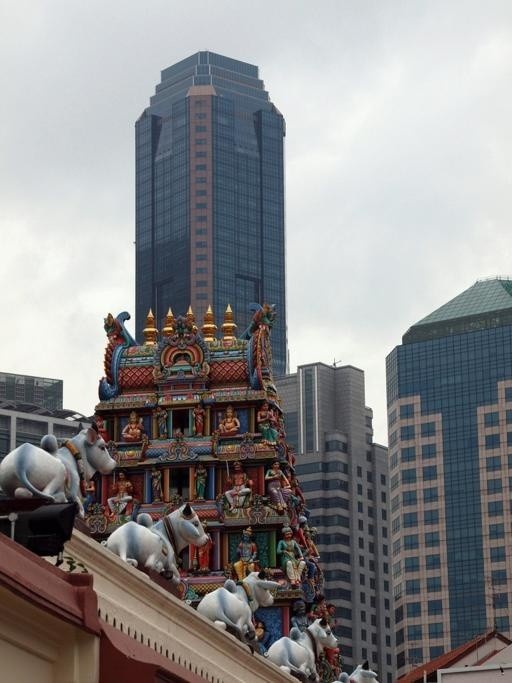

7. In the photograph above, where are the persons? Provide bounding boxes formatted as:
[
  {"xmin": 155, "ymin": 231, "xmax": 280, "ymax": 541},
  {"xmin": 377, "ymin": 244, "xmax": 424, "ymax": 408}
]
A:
[
  {"xmin": 196, "ymin": 521, "xmax": 212, "ymax": 574},
  {"xmin": 265, "ymin": 456, "xmax": 289, "ymax": 513},
  {"xmin": 278, "ymin": 518, "xmax": 343, "ymax": 682},
  {"xmin": 194, "ymin": 465, "xmax": 209, "ymax": 500},
  {"xmin": 191, "ymin": 400, "xmax": 207, "ymax": 438},
  {"xmin": 95, "ymin": 412, "xmax": 110, "ymax": 443},
  {"xmin": 120, "ymin": 411, "xmax": 145, "ymax": 442},
  {"xmin": 150, "ymin": 465, "xmax": 165, "ymax": 504},
  {"xmin": 86, "ymin": 478, "xmax": 98, "ymax": 512},
  {"xmin": 223, "ymin": 461, "xmax": 253, "ymax": 512},
  {"xmin": 257, "ymin": 401, "xmax": 278, "ymax": 443},
  {"xmin": 106, "ymin": 470, "xmax": 134, "ymax": 516},
  {"xmin": 218, "ymin": 405, "xmax": 240, "ymax": 435},
  {"xmin": 153, "ymin": 403, "xmax": 169, "ymax": 439},
  {"xmin": 232, "ymin": 526, "xmax": 258, "ymax": 580}
]
